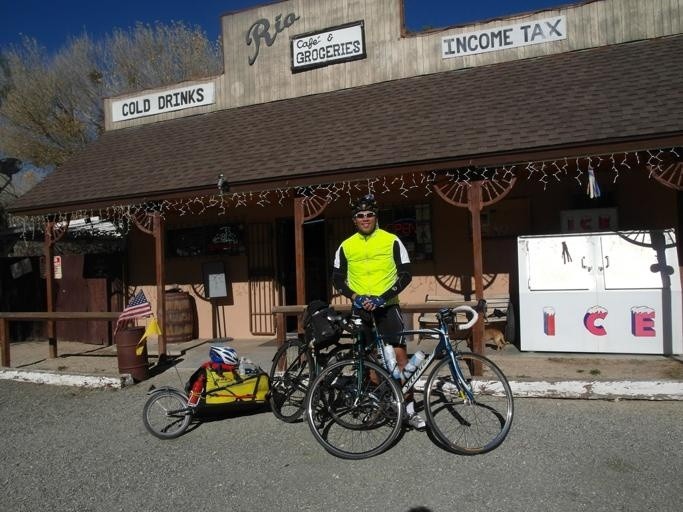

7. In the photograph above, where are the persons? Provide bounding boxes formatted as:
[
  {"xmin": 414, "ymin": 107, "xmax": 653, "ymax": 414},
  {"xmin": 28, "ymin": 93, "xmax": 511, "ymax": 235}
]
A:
[{"xmin": 333, "ymin": 196, "xmax": 426, "ymax": 432}]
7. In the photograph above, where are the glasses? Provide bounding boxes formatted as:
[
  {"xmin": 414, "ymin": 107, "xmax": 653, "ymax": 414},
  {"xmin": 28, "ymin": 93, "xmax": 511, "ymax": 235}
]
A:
[{"xmin": 354, "ymin": 212, "xmax": 375, "ymax": 217}]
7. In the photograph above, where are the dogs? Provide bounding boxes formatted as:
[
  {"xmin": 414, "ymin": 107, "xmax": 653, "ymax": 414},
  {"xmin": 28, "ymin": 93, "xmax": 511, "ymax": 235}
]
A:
[{"xmin": 465, "ymin": 328, "xmax": 510, "ymax": 351}]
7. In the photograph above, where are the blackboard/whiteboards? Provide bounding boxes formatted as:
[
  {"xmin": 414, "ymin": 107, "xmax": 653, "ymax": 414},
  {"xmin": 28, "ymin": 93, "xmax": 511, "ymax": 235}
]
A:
[{"xmin": 516, "ymin": 228, "xmax": 683, "ymax": 356}]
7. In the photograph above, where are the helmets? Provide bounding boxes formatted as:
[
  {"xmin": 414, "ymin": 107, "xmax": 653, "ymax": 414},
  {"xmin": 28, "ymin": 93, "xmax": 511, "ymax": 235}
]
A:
[
  {"xmin": 352, "ymin": 198, "xmax": 379, "ymax": 216},
  {"xmin": 210, "ymin": 346, "xmax": 239, "ymax": 366}
]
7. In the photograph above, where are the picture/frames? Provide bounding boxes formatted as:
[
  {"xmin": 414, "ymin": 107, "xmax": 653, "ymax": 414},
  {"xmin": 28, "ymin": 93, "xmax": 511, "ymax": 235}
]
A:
[{"xmin": 289, "ymin": 20, "xmax": 367, "ymax": 74}]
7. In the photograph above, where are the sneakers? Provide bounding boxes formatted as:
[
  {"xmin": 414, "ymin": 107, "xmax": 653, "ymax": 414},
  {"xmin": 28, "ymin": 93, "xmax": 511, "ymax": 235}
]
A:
[{"xmin": 408, "ymin": 412, "xmax": 426, "ymax": 428}]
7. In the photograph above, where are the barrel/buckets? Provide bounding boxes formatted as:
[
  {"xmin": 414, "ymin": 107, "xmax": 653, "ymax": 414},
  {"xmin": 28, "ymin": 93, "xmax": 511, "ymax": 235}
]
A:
[
  {"xmin": 164, "ymin": 290, "xmax": 193, "ymax": 344},
  {"xmin": 114, "ymin": 326, "xmax": 148, "ymax": 381}
]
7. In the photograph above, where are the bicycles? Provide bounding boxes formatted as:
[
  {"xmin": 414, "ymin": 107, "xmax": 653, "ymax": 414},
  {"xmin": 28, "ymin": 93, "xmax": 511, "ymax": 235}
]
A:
[
  {"xmin": 322, "ymin": 299, "xmax": 516, "ymax": 456},
  {"xmin": 140, "ymin": 359, "xmax": 273, "ymax": 442},
  {"xmin": 266, "ymin": 301, "xmax": 408, "ymax": 462}
]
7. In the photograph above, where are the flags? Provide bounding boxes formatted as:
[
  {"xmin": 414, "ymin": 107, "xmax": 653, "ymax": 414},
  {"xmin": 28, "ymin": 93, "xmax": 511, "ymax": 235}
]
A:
[{"xmin": 112, "ymin": 288, "xmax": 154, "ymax": 341}]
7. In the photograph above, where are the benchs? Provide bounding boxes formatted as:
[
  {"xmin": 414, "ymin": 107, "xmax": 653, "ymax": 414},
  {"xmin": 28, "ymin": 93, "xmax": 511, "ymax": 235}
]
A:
[{"xmin": 417, "ymin": 294, "xmax": 511, "ymax": 345}]
